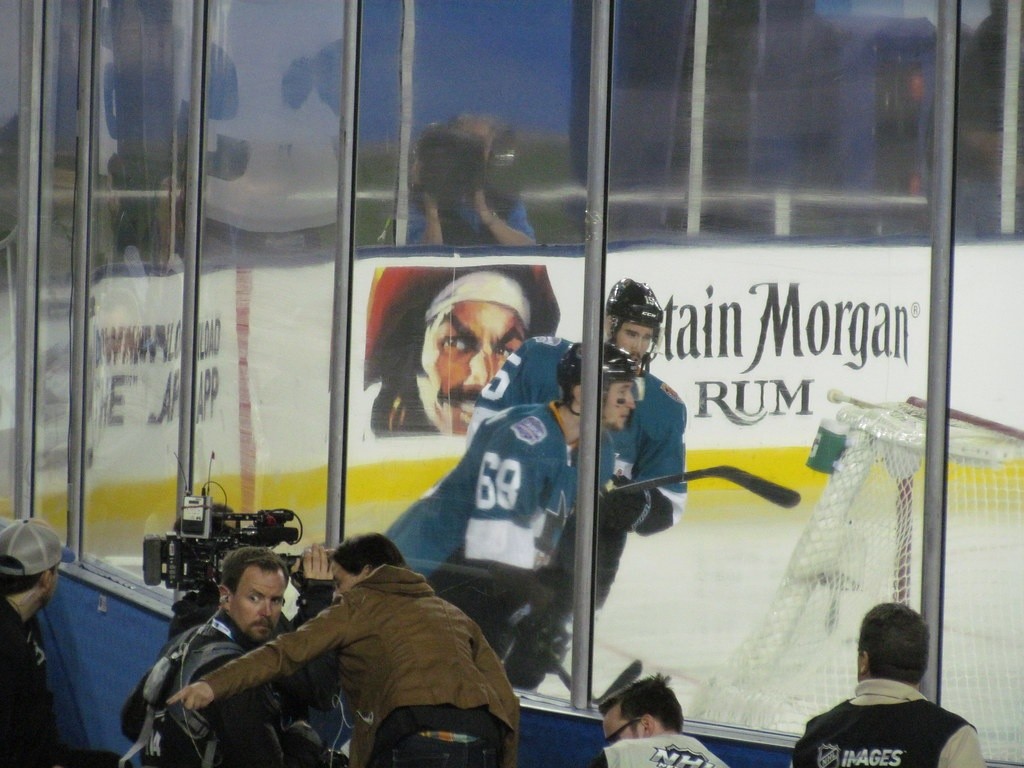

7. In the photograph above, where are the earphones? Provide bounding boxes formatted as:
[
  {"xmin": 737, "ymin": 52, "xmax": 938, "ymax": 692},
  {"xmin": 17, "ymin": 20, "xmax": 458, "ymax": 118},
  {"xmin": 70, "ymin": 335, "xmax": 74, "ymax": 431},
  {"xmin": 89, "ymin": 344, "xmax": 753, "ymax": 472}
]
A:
[{"xmin": 220, "ymin": 595, "xmax": 228, "ymax": 603}]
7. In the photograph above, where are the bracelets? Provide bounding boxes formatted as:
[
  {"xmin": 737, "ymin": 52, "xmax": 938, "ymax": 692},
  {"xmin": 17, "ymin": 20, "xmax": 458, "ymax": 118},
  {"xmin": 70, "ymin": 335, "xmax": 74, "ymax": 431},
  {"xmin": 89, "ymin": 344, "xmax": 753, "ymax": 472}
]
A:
[{"xmin": 482, "ymin": 212, "xmax": 498, "ymax": 229}]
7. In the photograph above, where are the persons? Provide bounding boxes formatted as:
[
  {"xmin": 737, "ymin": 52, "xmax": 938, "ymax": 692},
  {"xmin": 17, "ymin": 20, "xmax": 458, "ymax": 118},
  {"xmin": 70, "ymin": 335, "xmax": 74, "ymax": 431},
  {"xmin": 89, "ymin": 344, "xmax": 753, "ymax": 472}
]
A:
[
  {"xmin": 379, "ymin": 116, "xmax": 536, "ymax": 247},
  {"xmin": 387, "ymin": 345, "xmax": 634, "ymax": 691},
  {"xmin": 587, "ymin": 672, "xmax": 729, "ymax": 768},
  {"xmin": 960, "ymin": 0, "xmax": 1024, "ymax": 235},
  {"xmin": 122, "ymin": 505, "xmax": 349, "ymax": 768},
  {"xmin": 167, "ymin": 532, "xmax": 520, "ymax": 768},
  {"xmin": 0, "ymin": 115, "xmax": 187, "ymax": 286},
  {"xmin": 469, "ymin": 280, "xmax": 685, "ymax": 670},
  {"xmin": 0, "ymin": 519, "xmax": 132, "ymax": 768},
  {"xmin": 790, "ymin": 604, "xmax": 988, "ymax": 768}
]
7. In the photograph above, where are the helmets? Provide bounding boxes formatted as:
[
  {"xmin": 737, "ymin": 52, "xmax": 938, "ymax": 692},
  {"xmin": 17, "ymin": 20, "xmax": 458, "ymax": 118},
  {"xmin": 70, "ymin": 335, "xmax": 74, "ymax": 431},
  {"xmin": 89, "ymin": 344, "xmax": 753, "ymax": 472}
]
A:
[
  {"xmin": 607, "ymin": 278, "xmax": 666, "ymax": 343},
  {"xmin": 554, "ymin": 340, "xmax": 640, "ymax": 405}
]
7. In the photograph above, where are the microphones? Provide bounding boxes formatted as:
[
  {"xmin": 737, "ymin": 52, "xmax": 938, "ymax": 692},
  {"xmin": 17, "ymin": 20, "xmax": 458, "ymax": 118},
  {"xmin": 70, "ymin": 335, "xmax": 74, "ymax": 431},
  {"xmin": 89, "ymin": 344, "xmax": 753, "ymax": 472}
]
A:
[{"xmin": 256, "ymin": 511, "xmax": 294, "ymax": 524}]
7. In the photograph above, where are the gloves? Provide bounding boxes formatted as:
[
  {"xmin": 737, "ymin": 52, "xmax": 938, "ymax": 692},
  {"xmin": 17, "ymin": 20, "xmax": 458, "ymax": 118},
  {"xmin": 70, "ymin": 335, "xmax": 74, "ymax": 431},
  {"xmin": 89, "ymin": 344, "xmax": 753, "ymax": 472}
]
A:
[
  {"xmin": 596, "ymin": 471, "xmax": 674, "ymax": 536},
  {"xmin": 496, "ymin": 582, "xmax": 550, "ymax": 638}
]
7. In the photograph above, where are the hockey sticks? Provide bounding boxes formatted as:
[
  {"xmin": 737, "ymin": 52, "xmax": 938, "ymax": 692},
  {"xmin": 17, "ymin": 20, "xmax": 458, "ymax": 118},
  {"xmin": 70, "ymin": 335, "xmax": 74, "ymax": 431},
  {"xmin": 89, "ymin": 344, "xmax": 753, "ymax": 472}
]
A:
[
  {"xmin": 608, "ymin": 465, "xmax": 801, "ymax": 509},
  {"xmin": 551, "ymin": 658, "xmax": 643, "ymax": 706}
]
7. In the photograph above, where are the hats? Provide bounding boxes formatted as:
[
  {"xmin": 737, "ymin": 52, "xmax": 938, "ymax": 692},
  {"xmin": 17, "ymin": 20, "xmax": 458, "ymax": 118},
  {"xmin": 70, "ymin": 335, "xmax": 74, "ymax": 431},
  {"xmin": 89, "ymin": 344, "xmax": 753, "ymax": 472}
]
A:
[{"xmin": 0, "ymin": 518, "xmax": 76, "ymax": 576}]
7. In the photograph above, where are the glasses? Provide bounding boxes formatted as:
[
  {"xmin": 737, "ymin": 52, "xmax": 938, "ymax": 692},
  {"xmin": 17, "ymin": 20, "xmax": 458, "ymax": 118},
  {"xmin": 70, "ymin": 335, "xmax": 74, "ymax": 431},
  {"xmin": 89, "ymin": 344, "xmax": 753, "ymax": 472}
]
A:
[{"xmin": 604, "ymin": 717, "xmax": 642, "ymax": 745}]
